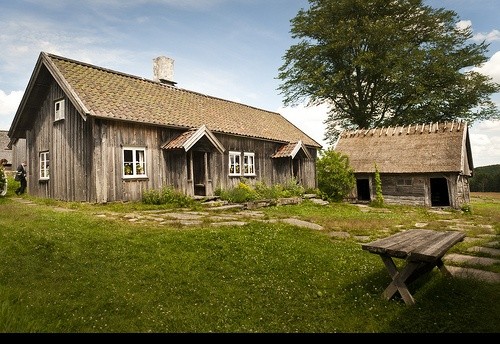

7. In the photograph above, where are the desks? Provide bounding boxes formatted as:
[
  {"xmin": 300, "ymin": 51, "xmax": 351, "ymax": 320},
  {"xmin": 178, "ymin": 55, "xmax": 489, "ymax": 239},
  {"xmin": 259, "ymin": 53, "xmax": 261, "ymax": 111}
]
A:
[{"xmin": 361, "ymin": 228, "xmax": 466, "ymax": 309}]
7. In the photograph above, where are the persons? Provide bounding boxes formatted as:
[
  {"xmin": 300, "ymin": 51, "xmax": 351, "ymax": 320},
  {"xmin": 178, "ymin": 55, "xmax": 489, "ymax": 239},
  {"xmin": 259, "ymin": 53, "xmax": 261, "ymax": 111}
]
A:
[
  {"xmin": 15, "ymin": 161, "xmax": 27, "ymax": 195},
  {"xmin": 0, "ymin": 159, "xmax": 9, "ymax": 196}
]
